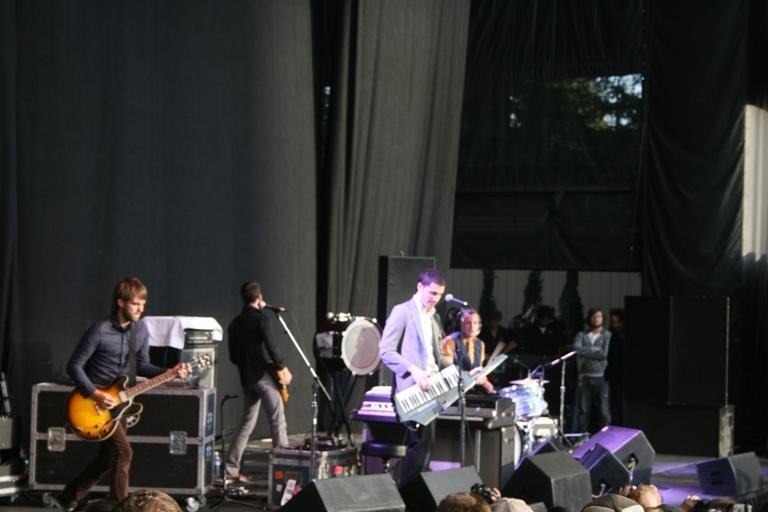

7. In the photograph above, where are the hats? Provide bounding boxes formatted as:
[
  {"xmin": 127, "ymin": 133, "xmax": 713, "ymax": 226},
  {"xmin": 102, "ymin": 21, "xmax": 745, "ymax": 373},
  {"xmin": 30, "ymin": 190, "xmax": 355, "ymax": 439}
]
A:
[
  {"xmin": 627, "ymin": 485, "xmax": 662, "ymax": 508},
  {"xmin": 581, "ymin": 493, "xmax": 644, "ymax": 512},
  {"xmin": 491, "ymin": 497, "xmax": 534, "ymax": 512}
]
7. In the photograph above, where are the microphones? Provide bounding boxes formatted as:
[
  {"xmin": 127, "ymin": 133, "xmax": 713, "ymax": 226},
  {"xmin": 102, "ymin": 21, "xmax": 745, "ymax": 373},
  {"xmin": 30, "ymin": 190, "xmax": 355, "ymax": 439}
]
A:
[
  {"xmin": 261, "ymin": 302, "xmax": 285, "ymax": 313},
  {"xmin": 327, "ymin": 312, "xmax": 344, "ymax": 319},
  {"xmin": 444, "ymin": 293, "xmax": 469, "ymax": 310}
]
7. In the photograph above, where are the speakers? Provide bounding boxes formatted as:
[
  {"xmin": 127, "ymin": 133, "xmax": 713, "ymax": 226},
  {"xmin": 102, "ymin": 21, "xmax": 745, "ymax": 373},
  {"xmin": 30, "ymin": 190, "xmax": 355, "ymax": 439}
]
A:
[
  {"xmin": 571, "ymin": 426, "xmax": 656, "ymax": 492},
  {"xmin": 277, "ymin": 472, "xmax": 409, "ymax": 512},
  {"xmin": 696, "ymin": 451, "xmax": 762, "ymax": 498},
  {"xmin": 501, "ymin": 451, "xmax": 593, "ymax": 512},
  {"xmin": 397, "ymin": 466, "xmax": 485, "ymax": 511},
  {"xmin": 516, "ymin": 431, "xmax": 566, "ymax": 467},
  {"xmin": 578, "ymin": 443, "xmax": 628, "ymax": 501}
]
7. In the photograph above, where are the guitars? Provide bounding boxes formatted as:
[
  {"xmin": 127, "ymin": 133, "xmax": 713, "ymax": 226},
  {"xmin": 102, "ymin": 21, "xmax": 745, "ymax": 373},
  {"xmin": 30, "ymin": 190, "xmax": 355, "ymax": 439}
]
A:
[{"xmin": 64, "ymin": 355, "xmax": 211, "ymax": 442}]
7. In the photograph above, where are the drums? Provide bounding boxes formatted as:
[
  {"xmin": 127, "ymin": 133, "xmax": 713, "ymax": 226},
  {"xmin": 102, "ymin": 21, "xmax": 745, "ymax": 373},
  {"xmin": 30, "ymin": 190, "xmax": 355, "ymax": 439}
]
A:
[
  {"xmin": 332, "ymin": 317, "xmax": 383, "ymax": 378},
  {"xmin": 499, "ymin": 382, "xmax": 558, "ymax": 470}
]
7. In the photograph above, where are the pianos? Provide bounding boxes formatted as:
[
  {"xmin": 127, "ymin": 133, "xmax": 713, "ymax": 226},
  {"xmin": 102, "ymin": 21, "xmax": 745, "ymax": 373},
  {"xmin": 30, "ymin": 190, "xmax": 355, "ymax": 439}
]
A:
[{"xmin": 354, "ymin": 385, "xmax": 515, "ymax": 430}]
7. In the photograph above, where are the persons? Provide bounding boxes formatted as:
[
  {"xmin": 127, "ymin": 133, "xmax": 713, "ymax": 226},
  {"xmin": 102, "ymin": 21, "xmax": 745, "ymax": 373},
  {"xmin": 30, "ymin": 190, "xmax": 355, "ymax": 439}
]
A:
[
  {"xmin": 476, "ymin": 300, "xmax": 573, "ymax": 392},
  {"xmin": 572, "ymin": 307, "xmax": 614, "ymax": 446},
  {"xmin": 434, "ymin": 483, "xmax": 768, "ymax": 512},
  {"xmin": 441, "ymin": 308, "xmax": 497, "ymax": 399},
  {"xmin": 379, "ymin": 270, "xmax": 446, "ymax": 489},
  {"xmin": 58, "ymin": 275, "xmax": 193, "ymax": 511},
  {"xmin": 111, "ymin": 488, "xmax": 183, "ymax": 512},
  {"xmin": 603, "ymin": 310, "xmax": 625, "ymax": 426},
  {"xmin": 223, "ymin": 280, "xmax": 294, "ymax": 481}
]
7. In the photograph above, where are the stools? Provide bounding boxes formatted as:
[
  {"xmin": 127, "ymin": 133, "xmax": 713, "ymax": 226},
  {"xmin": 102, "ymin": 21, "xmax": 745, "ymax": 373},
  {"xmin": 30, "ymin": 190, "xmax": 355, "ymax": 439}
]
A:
[{"xmin": 361, "ymin": 441, "xmax": 408, "ymax": 474}]
